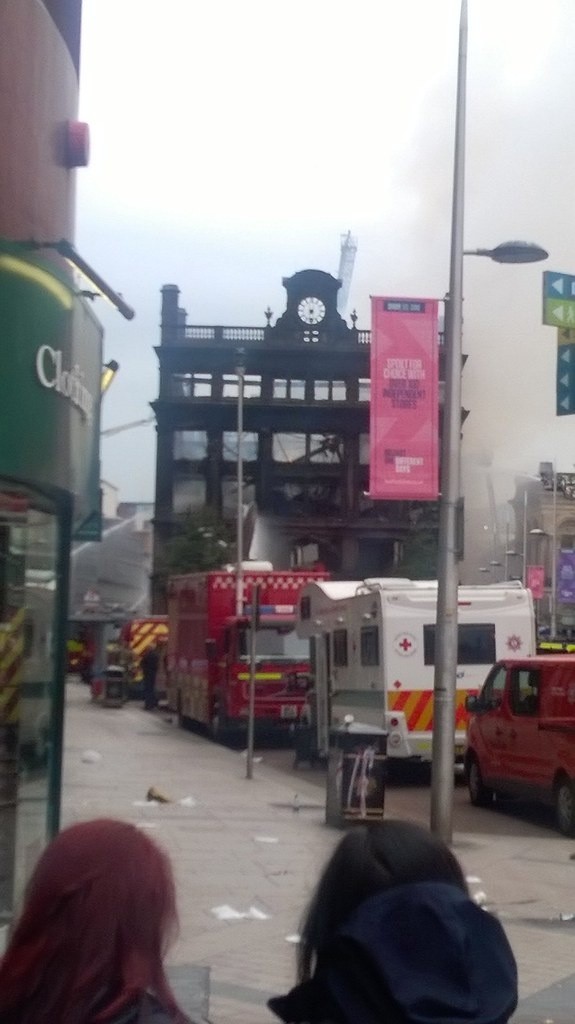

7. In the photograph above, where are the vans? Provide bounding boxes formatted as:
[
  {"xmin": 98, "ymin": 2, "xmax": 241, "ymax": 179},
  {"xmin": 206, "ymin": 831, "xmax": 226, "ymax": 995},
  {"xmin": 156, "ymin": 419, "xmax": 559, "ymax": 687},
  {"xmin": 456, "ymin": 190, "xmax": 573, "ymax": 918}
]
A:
[{"xmin": 467, "ymin": 657, "xmax": 575, "ymax": 836}]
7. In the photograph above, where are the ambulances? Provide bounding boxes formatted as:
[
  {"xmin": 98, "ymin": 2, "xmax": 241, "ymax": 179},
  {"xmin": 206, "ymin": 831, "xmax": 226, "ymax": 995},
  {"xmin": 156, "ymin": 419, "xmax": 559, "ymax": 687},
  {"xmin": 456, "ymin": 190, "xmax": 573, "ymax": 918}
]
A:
[{"xmin": 288, "ymin": 578, "xmax": 536, "ymax": 797}]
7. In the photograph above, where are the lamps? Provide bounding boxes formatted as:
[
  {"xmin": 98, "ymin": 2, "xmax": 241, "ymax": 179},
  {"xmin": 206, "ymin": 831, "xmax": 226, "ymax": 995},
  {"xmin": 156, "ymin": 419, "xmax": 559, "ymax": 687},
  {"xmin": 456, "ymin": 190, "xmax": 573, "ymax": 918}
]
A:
[{"xmin": 102, "ymin": 358, "xmax": 120, "ymax": 374}]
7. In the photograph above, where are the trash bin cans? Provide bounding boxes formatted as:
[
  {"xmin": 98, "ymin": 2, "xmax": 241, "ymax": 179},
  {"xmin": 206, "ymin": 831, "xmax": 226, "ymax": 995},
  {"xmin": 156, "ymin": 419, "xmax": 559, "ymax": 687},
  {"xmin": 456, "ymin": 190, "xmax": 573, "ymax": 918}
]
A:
[
  {"xmin": 102, "ymin": 664, "xmax": 125, "ymax": 709},
  {"xmin": 323, "ymin": 721, "xmax": 392, "ymax": 831}
]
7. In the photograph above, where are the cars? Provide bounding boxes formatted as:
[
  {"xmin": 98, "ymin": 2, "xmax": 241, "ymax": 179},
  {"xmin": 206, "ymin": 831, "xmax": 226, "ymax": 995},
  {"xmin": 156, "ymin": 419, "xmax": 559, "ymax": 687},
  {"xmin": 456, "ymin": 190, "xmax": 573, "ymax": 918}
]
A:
[{"xmin": 67, "ymin": 614, "xmax": 169, "ymax": 699}]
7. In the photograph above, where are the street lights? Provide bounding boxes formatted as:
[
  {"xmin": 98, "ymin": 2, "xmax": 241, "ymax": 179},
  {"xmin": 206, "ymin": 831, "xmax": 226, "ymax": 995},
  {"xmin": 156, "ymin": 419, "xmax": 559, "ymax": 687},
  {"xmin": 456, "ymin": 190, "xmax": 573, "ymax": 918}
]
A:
[{"xmin": 420, "ymin": 2, "xmax": 548, "ymax": 853}]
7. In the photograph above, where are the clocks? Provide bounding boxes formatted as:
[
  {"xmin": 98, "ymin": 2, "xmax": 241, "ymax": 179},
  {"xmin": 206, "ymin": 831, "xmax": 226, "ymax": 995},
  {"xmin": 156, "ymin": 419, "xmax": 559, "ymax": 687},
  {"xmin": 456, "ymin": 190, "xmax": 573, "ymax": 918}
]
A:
[{"xmin": 297, "ymin": 297, "xmax": 326, "ymax": 325}]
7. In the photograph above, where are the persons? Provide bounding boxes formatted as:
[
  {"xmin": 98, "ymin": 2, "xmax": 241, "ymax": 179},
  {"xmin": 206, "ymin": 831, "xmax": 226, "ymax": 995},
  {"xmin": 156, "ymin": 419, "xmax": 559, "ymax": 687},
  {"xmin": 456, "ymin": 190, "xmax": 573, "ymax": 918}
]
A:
[
  {"xmin": 266, "ymin": 821, "xmax": 519, "ymax": 1024},
  {"xmin": 0, "ymin": 821, "xmax": 195, "ymax": 1024},
  {"xmin": 139, "ymin": 647, "xmax": 161, "ymax": 710}
]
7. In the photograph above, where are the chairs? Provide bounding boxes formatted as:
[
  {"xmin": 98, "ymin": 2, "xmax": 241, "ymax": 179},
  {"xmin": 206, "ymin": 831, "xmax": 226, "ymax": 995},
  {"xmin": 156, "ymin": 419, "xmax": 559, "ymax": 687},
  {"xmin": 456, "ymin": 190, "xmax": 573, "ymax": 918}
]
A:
[{"xmin": 516, "ymin": 694, "xmax": 537, "ymax": 716}]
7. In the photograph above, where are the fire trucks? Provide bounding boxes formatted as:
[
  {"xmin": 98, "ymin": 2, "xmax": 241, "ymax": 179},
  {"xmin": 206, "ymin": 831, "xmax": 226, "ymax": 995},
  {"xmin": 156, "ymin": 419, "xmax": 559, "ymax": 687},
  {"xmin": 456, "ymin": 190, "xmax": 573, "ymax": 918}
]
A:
[{"xmin": 166, "ymin": 573, "xmax": 329, "ymax": 742}]
